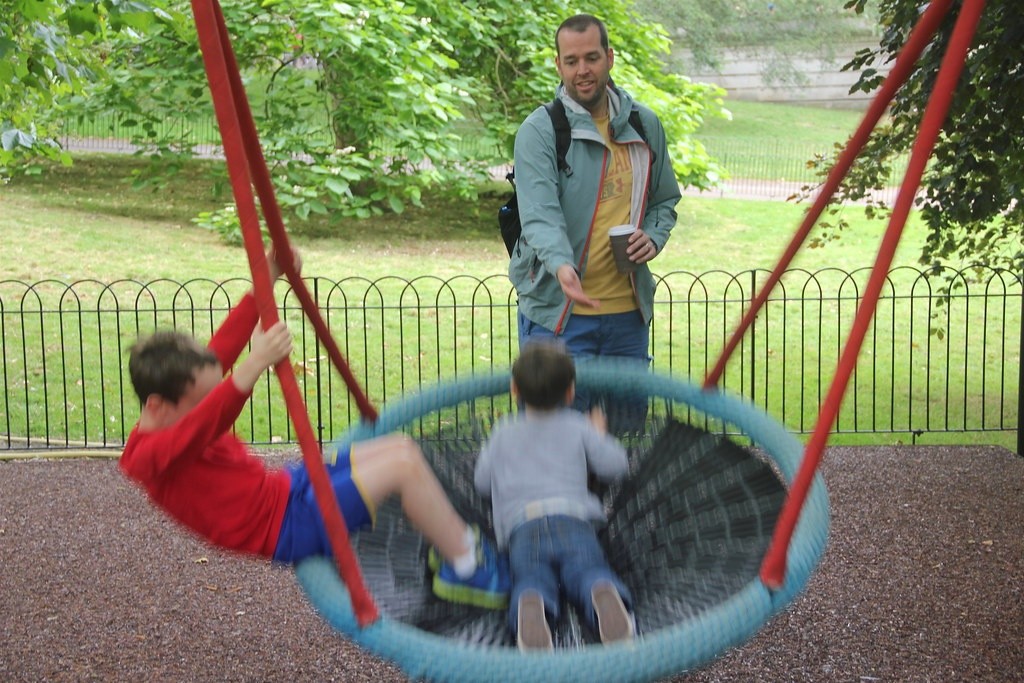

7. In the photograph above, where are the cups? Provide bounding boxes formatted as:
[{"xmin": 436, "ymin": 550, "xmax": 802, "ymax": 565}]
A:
[{"xmin": 608, "ymin": 223, "xmax": 640, "ymax": 273}]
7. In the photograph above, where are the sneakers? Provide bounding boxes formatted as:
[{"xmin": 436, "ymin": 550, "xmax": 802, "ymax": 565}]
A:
[
  {"xmin": 433, "ymin": 551, "xmax": 511, "ymax": 610},
  {"xmin": 428, "ymin": 523, "xmax": 510, "ymax": 572}
]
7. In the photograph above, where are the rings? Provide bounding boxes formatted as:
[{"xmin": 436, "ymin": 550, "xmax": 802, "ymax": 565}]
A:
[{"xmin": 645, "ymin": 242, "xmax": 651, "ymax": 249}]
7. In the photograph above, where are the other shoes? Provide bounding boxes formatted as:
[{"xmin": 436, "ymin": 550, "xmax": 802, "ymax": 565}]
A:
[
  {"xmin": 516, "ymin": 588, "xmax": 552, "ymax": 653},
  {"xmin": 591, "ymin": 577, "xmax": 634, "ymax": 644}
]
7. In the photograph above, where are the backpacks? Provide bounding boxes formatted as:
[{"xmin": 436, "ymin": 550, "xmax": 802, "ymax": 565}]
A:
[{"xmin": 498, "ymin": 96, "xmax": 644, "ymax": 260}]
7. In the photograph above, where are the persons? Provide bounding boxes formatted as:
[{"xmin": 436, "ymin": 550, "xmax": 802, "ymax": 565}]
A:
[
  {"xmin": 119, "ymin": 239, "xmax": 510, "ymax": 609},
  {"xmin": 473, "ymin": 339, "xmax": 637, "ymax": 651},
  {"xmin": 509, "ymin": 13, "xmax": 682, "ymax": 440}
]
